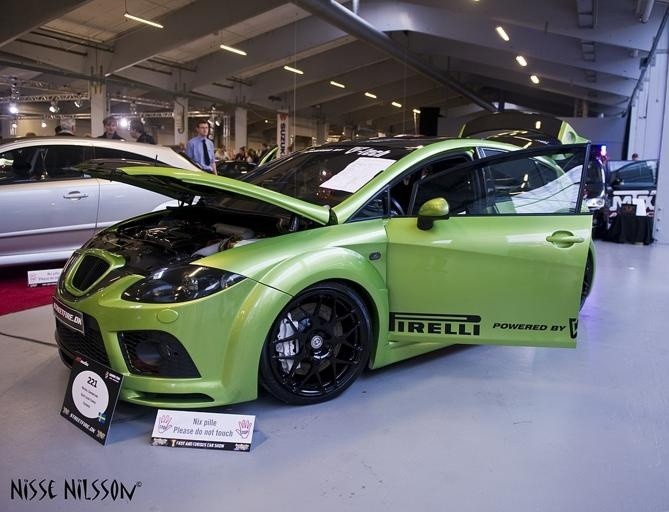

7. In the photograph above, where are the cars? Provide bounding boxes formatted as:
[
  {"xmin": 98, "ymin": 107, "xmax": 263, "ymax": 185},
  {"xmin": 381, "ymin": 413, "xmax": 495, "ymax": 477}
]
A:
[
  {"xmin": 206, "ymin": 159, "xmax": 258, "ymax": 181},
  {"xmin": 0, "ymin": 139, "xmax": 208, "ymax": 272},
  {"xmin": 570, "ymin": 154, "xmax": 659, "ymax": 244},
  {"xmin": 48, "ymin": 105, "xmax": 591, "ymax": 413}
]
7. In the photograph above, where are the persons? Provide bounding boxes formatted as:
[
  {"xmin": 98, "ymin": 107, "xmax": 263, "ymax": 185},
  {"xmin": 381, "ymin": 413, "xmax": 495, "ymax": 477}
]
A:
[
  {"xmin": 586, "ymin": 145, "xmax": 613, "ymax": 232},
  {"xmin": 128, "ymin": 119, "xmax": 155, "ymax": 146},
  {"xmin": 54, "ymin": 116, "xmax": 76, "ymax": 137},
  {"xmin": 186, "ymin": 120, "xmax": 218, "ymax": 175},
  {"xmin": 214, "ymin": 140, "xmax": 275, "ymax": 166},
  {"xmin": 631, "ymin": 153, "xmax": 640, "ymax": 161},
  {"xmin": 96, "ymin": 115, "xmax": 125, "ymax": 140}
]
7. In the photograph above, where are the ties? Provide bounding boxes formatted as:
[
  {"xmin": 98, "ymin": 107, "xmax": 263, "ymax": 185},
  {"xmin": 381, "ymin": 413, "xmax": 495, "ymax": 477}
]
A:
[{"xmin": 202, "ymin": 139, "xmax": 210, "ymax": 165}]
